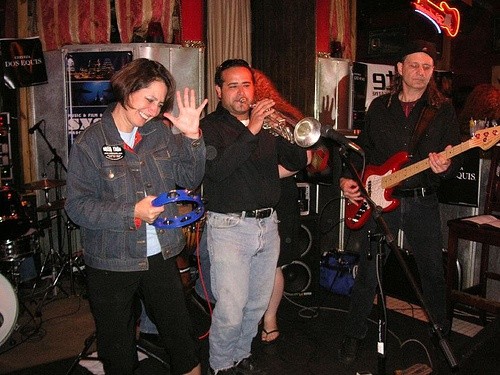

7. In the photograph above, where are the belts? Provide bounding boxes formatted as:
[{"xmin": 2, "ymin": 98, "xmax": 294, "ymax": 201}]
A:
[
  {"xmin": 228, "ymin": 209, "xmax": 271, "ymax": 219},
  {"xmin": 391, "ymin": 186, "xmax": 436, "ymax": 199}
]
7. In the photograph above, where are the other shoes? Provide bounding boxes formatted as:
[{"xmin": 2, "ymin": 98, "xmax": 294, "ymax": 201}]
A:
[
  {"xmin": 239, "ymin": 357, "xmax": 271, "ymax": 375},
  {"xmin": 430, "ymin": 333, "xmax": 454, "ymax": 362},
  {"xmin": 209, "ymin": 365, "xmax": 248, "ymax": 375},
  {"xmin": 337, "ymin": 334, "xmax": 359, "ymax": 366}
]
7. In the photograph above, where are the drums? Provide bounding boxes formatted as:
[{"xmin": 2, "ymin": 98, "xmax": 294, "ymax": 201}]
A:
[
  {"xmin": 0, "ymin": 272, "xmax": 20, "ymax": 347},
  {"xmin": 0, "ymin": 185, "xmax": 31, "ymax": 240},
  {"xmin": 0, "ymin": 234, "xmax": 37, "ymax": 262}
]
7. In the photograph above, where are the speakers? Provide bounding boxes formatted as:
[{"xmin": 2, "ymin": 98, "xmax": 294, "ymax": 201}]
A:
[{"xmin": 277, "ymin": 212, "xmax": 322, "ymax": 301}]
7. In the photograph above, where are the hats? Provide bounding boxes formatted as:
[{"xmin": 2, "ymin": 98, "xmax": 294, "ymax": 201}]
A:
[{"xmin": 399, "ymin": 39, "xmax": 437, "ymax": 66}]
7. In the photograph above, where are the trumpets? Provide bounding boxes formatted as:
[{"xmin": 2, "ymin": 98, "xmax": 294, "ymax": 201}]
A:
[{"xmin": 238, "ymin": 98, "xmax": 321, "ymax": 147}]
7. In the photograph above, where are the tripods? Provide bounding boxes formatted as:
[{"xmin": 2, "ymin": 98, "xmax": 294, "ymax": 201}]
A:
[{"xmin": 28, "ymin": 191, "xmax": 88, "ymax": 319}]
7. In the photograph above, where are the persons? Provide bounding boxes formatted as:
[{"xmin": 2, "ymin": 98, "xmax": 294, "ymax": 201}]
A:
[
  {"xmin": 137, "ymin": 57, "xmax": 312, "ymax": 375},
  {"xmin": 339, "ymin": 40, "xmax": 461, "ymax": 363},
  {"xmin": 63, "ymin": 58, "xmax": 209, "ymax": 375}
]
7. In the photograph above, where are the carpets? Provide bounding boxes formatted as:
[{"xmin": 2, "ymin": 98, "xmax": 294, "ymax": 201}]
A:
[{"xmin": 11, "ymin": 287, "xmax": 470, "ymax": 375}]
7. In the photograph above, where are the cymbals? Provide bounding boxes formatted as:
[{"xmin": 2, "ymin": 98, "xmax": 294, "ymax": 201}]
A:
[
  {"xmin": 20, "ymin": 180, "xmax": 67, "ymax": 190},
  {"xmin": 33, "ymin": 198, "xmax": 67, "ymax": 212},
  {"xmin": 151, "ymin": 188, "xmax": 206, "ymax": 229}
]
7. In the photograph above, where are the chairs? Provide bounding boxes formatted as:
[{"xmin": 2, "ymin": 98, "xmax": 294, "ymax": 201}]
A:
[{"xmin": 447, "ymin": 142, "xmax": 500, "ymax": 331}]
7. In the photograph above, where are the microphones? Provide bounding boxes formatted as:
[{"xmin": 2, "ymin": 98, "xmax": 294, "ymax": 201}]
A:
[
  {"xmin": 28, "ymin": 120, "xmax": 43, "ymax": 134},
  {"xmin": 320, "ymin": 124, "xmax": 365, "ymax": 157}
]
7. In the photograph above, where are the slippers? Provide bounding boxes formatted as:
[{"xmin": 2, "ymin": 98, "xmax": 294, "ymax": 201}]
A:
[{"xmin": 261, "ymin": 323, "xmax": 281, "ymax": 345}]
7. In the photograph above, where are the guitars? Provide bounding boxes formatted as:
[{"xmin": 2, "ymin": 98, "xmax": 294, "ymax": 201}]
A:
[{"xmin": 344, "ymin": 122, "xmax": 500, "ymax": 228}]
